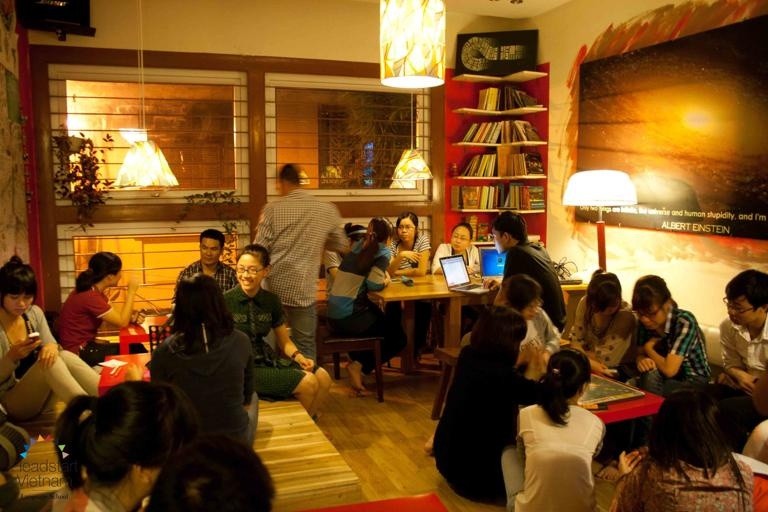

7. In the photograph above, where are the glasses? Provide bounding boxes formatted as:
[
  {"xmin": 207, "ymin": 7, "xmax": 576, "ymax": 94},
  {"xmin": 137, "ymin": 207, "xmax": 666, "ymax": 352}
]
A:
[
  {"xmin": 631, "ymin": 296, "xmax": 669, "ymax": 320},
  {"xmin": 722, "ymin": 296, "xmax": 760, "ymax": 315},
  {"xmin": 236, "ymin": 267, "xmax": 266, "ymax": 274}
]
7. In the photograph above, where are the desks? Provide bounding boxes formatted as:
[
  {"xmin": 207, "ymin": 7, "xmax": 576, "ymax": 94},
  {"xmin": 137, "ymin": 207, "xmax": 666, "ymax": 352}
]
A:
[
  {"xmin": 728, "ymin": 452, "xmax": 768, "ymax": 510},
  {"xmin": 310, "ymin": 493, "xmax": 449, "ymax": 510},
  {"xmin": 360, "ymin": 270, "xmax": 594, "ymax": 373},
  {"xmin": 573, "ymin": 373, "xmax": 665, "ymax": 461},
  {"xmin": 95, "ymin": 314, "xmax": 174, "ymax": 400}
]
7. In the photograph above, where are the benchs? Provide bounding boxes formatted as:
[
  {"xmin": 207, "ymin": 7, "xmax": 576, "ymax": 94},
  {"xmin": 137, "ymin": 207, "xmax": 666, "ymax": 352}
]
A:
[{"xmin": 431, "ymin": 339, "xmax": 570, "ymax": 420}]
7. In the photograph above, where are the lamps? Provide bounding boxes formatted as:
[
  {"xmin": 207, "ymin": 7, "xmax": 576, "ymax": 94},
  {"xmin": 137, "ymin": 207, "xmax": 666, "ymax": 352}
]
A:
[
  {"xmin": 562, "ymin": 167, "xmax": 635, "ymax": 274},
  {"xmin": 390, "ymin": 86, "xmax": 433, "ymax": 181},
  {"xmin": 375, "ymin": 0, "xmax": 445, "ymax": 88},
  {"xmin": 109, "ymin": 0, "xmax": 180, "ymax": 188}
]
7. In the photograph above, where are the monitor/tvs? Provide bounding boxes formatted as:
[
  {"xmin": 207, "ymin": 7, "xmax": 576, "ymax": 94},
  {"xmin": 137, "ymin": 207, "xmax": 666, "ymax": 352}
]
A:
[{"xmin": 14, "ymin": 0, "xmax": 91, "ymax": 27}]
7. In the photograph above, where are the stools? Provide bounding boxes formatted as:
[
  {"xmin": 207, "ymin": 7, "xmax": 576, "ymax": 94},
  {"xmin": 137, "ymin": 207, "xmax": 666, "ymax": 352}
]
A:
[{"xmin": 316, "ymin": 325, "xmax": 384, "ymax": 406}]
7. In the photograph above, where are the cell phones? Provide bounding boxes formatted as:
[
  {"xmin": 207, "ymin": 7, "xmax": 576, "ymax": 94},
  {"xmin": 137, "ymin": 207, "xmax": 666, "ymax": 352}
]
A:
[
  {"xmin": 134, "ymin": 307, "xmax": 147, "ymax": 324},
  {"xmin": 28, "ymin": 331, "xmax": 40, "ymax": 345}
]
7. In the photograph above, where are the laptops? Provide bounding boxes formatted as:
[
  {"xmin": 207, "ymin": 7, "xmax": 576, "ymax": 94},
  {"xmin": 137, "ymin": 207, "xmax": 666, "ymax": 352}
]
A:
[
  {"xmin": 478, "ymin": 246, "xmax": 507, "ymax": 280},
  {"xmin": 439, "ymin": 254, "xmax": 490, "ymax": 295}
]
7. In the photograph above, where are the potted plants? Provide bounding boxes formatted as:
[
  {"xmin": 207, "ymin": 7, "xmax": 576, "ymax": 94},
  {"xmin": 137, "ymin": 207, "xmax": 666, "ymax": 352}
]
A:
[{"xmin": 48, "ymin": 125, "xmax": 116, "ymax": 233}]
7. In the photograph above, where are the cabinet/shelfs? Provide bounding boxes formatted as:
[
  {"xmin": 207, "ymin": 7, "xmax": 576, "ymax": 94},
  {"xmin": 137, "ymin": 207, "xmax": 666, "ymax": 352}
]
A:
[{"xmin": 445, "ymin": 67, "xmax": 549, "ymax": 248}]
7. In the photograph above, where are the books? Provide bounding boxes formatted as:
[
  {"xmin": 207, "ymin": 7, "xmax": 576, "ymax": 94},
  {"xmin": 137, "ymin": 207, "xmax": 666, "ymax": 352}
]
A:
[{"xmin": 459, "ymin": 85, "xmax": 546, "ymax": 211}]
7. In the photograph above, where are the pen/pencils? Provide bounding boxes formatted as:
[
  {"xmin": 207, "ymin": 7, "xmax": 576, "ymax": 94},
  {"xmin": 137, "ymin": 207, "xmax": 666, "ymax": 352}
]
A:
[{"xmin": 202, "ymin": 323, "xmax": 209, "ymax": 353}]
